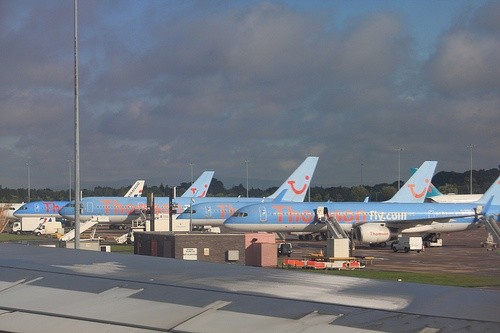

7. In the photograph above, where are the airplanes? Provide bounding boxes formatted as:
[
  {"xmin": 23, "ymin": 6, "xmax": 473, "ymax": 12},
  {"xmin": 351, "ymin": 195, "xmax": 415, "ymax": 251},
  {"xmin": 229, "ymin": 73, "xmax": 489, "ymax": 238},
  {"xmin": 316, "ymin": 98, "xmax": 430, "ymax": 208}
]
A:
[{"xmin": 0, "ymin": 156, "xmax": 500, "ymax": 247}]
